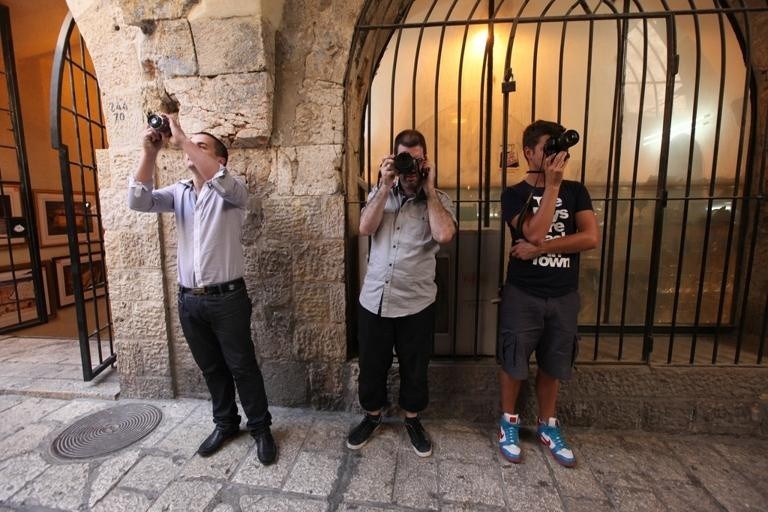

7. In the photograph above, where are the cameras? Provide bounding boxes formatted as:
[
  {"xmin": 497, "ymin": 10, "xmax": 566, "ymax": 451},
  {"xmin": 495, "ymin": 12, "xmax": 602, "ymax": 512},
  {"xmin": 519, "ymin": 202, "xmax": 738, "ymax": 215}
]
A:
[
  {"xmin": 543, "ymin": 129, "xmax": 580, "ymax": 162},
  {"xmin": 393, "ymin": 150, "xmax": 419, "ymax": 175},
  {"xmin": 147, "ymin": 114, "xmax": 169, "ymax": 132}
]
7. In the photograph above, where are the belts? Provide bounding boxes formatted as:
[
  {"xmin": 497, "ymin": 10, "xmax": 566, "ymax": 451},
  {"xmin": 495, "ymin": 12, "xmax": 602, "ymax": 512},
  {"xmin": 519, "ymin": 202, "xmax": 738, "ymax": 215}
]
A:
[{"xmin": 179, "ymin": 278, "xmax": 244, "ymax": 295}]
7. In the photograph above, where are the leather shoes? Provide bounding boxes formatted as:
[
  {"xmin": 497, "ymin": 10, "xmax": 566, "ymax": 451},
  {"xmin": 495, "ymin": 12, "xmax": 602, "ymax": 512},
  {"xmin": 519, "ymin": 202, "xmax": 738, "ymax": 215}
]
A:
[
  {"xmin": 198, "ymin": 424, "xmax": 240, "ymax": 455},
  {"xmin": 253, "ymin": 427, "xmax": 277, "ymax": 464}
]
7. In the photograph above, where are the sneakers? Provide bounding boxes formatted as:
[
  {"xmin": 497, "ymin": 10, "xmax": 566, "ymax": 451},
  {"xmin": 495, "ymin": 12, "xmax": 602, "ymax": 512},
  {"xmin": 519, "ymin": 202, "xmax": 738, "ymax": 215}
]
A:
[
  {"xmin": 345, "ymin": 411, "xmax": 383, "ymax": 450},
  {"xmin": 498, "ymin": 411, "xmax": 523, "ymax": 464},
  {"xmin": 403, "ymin": 415, "xmax": 433, "ymax": 457},
  {"xmin": 536, "ymin": 415, "xmax": 578, "ymax": 468}
]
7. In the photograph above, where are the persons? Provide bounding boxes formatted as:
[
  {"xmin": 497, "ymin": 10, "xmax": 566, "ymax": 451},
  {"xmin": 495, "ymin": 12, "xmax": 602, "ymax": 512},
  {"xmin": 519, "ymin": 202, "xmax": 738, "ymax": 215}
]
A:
[
  {"xmin": 498, "ymin": 120, "xmax": 600, "ymax": 467},
  {"xmin": 343, "ymin": 131, "xmax": 458, "ymax": 459},
  {"xmin": 125, "ymin": 112, "xmax": 280, "ymax": 469}
]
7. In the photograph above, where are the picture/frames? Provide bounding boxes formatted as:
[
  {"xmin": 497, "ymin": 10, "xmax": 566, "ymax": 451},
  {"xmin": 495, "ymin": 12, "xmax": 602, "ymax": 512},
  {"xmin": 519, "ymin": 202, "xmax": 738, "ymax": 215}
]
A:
[
  {"xmin": 51, "ymin": 253, "xmax": 106, "ymax": 308},
  {"xmin": 31, "ymin": 187, "xmax": 104, "ymax": 247},
  {"xmin": 0, "ymin": 260, "xmax": 56, "ymax": 336},
  {"xmin": 0, "ymin": 186, "xmax": 32, "ymax": 250}
]
